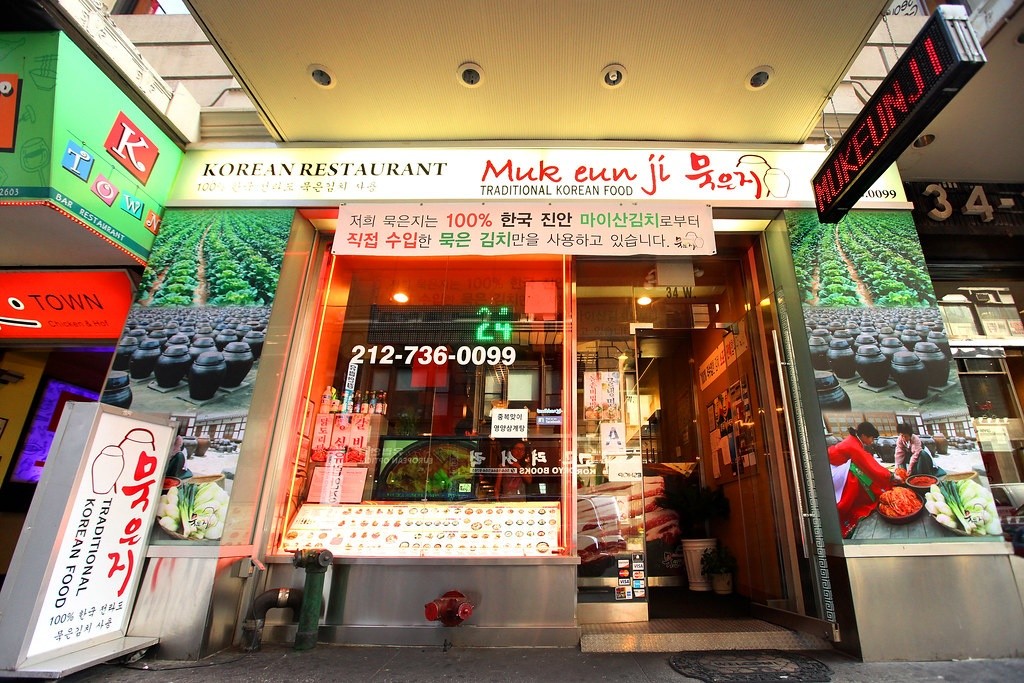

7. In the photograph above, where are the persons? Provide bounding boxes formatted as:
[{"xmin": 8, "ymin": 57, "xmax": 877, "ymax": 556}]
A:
[
  {"xmin": 494, "ymin": 442, "xmax": 533, "ymax": 501},
  {"xmin": 713, "ymin": 390, "xmax": 731, "ymax": 426},
  {"xmin": 895, "ymin": 423, "xmax": 946, "ymax": 481},
  {"xmin": 827, "ymin": 422, "xmax": 894, "ymax": 539}
]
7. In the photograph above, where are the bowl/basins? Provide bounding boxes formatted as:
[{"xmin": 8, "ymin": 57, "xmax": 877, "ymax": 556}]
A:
[
  {"xmin": 399, "ymin": 508, "xmax": 556, "ymax": 552},
  {"xmin": 906, "ymin": 473, "xmax": 938, "ymax": 497},
  {"xmin": 878, "ymin": 489, "xmax": 924, "ymax": 525}
]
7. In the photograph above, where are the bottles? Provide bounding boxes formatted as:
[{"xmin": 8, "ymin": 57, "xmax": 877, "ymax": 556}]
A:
[{"xmin": 320, "ymin": 386, "xmax": 387, "ymax": 415}]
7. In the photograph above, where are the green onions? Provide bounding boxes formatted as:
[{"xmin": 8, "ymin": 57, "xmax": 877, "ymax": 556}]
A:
[
  {"xmin": 177, "ymin": 481, "xmax": 200, "ymax": 536},
  {"xmin": 937, "ymin": 480, "xmax": 978, "ymax": 534}
]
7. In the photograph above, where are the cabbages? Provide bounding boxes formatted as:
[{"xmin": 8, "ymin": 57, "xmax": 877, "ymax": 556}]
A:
[
  {"xmin": 958, "ymin": 478, "xmax": 1002, "ymax": 536},
  {"xmin": 155, "ymin": 485, "xmax": 180, "ymax": 532},
  {"xmin": 925, "ymin": 484, "xmax": 960, "ymax": 529},
  {"xmin": 194, "ymin": 482, "xmax": 230, "ymax": 538}
]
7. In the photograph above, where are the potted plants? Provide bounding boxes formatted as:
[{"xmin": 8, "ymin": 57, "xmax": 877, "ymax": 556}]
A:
[{"xmin": 658, "ymin": 482, "xmax": 738, "ymax": 595}]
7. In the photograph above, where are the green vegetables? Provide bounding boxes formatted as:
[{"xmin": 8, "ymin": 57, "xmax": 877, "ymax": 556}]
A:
[{"xmin": 387, "ymin": 448, "xmax": 475, "ymax": 493}]
